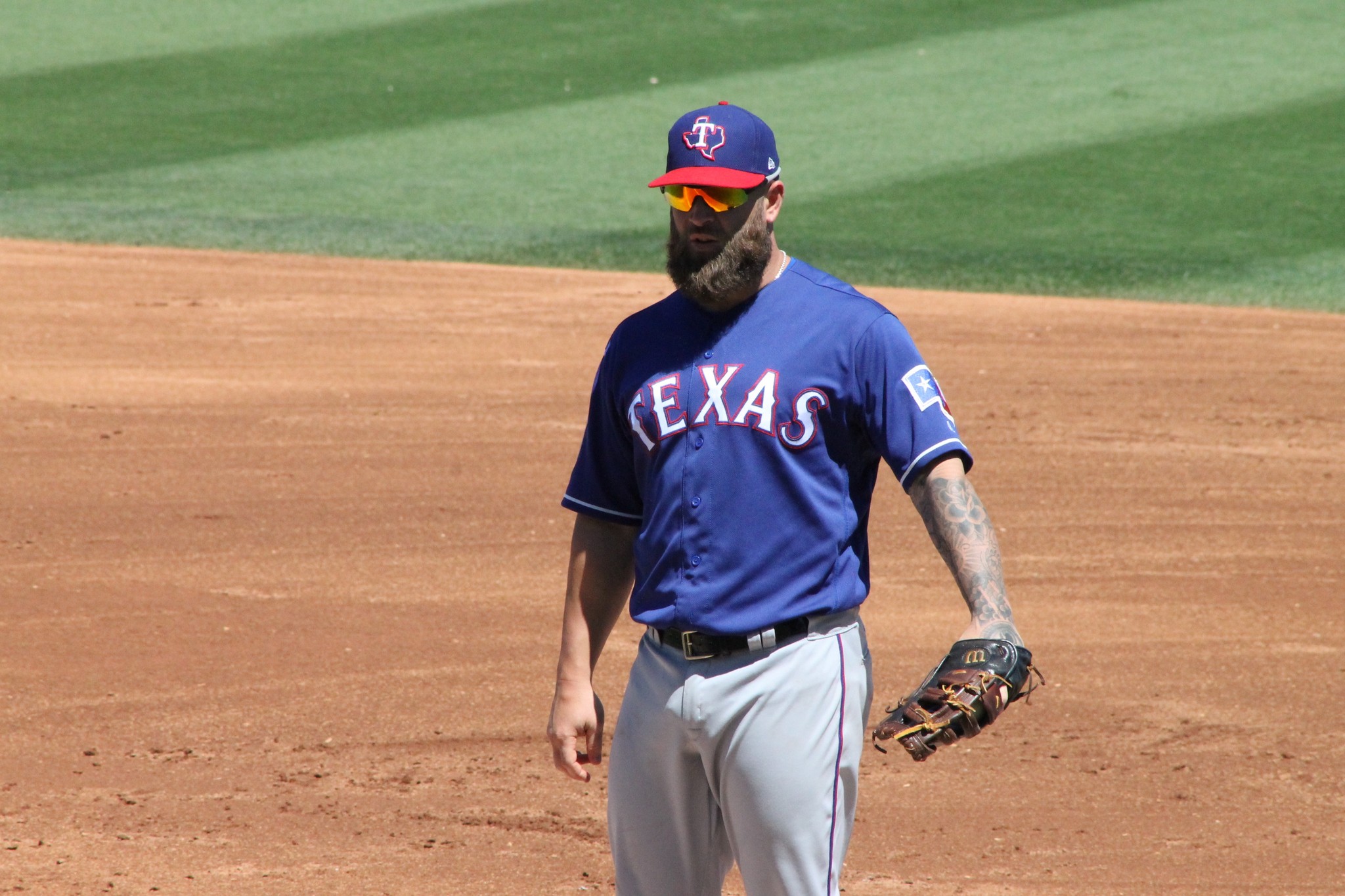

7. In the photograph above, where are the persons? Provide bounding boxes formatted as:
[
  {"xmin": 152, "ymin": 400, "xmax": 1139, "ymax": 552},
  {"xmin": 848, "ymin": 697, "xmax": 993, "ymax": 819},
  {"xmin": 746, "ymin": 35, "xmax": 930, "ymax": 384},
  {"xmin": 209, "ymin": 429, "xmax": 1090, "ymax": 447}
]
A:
[{"xmin": 546, "ymin": 100, "xmax": 1032, "ymax": 896}]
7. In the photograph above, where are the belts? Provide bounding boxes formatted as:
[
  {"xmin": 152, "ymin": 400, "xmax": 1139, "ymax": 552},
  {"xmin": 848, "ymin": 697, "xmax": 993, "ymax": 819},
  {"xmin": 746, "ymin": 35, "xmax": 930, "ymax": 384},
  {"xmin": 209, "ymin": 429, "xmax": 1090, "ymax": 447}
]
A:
[{"xmin": 649, "ymin": 618, "xmax": 809, "ymax": 661}]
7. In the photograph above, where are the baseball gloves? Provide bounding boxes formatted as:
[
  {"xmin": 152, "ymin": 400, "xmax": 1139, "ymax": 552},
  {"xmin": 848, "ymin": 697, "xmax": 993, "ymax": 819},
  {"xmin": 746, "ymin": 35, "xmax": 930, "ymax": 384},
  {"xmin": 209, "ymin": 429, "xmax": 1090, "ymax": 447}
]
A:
[{"xmin": 871, "ymin": 630, "xmax": 1032, "ymax": 763}]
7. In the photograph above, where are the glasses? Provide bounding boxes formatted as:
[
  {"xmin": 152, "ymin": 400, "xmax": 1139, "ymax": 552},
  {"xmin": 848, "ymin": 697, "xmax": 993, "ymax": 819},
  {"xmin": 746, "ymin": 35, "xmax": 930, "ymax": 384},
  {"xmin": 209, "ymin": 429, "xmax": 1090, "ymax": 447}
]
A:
[{"xmin": 660, "ymin": 168, "xmax": 782, "ymax": 212}]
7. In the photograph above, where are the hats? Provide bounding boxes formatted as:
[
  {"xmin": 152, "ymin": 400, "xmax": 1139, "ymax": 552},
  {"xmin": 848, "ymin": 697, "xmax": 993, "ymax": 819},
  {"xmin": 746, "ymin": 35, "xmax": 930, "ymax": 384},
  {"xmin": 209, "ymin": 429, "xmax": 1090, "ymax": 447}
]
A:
[{"xmin": 649, "ymin": 101, "xmax": 779, "ymax": 189}]
